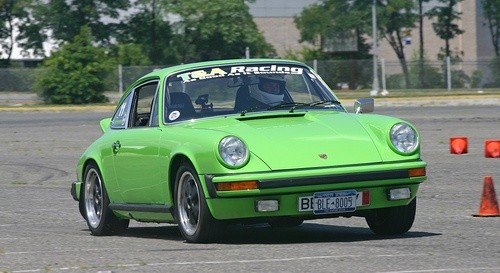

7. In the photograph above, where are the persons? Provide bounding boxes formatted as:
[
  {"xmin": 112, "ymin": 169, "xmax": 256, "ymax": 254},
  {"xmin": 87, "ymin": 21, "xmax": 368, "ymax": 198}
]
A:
[{"xmin": 248, "ymin": 75, "xmax": 286, "ymax": 112}]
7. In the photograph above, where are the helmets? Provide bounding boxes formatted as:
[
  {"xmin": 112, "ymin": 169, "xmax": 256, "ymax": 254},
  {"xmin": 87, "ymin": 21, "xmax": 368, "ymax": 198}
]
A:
[{"xmin": 247, "ymin": 71, "xmax": 287, "ymax": 106}]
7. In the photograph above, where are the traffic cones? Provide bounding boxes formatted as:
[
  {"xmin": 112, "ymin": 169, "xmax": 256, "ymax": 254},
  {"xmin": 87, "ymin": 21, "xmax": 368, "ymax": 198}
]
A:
[
  {"xmin": 484, "ymin": 140, "xmax": 500, "ymax": 158},
  {"xmin": 449, "ymin": 137, "xmax": 467, "ymax": 154},
  {"xmin": 471, "ymin": 176, "xmax": 500, "ymax": 217}
]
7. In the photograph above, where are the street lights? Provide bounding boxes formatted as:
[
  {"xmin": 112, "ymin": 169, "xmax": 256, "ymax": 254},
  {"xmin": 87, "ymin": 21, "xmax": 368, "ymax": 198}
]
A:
[{"xmin": 347, "ymin": 0, "xmax": 387, "ymax": 96}]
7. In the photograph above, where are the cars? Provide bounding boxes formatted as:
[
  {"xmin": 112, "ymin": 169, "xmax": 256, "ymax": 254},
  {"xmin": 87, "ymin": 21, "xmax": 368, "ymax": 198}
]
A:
[
  {"xmin": 336, "ymin": 79, "xmax": 357, "ymax": 91},
  {"xmin": 69, "ymin": 58, "xmax": 426, "ymax": 243}
]
